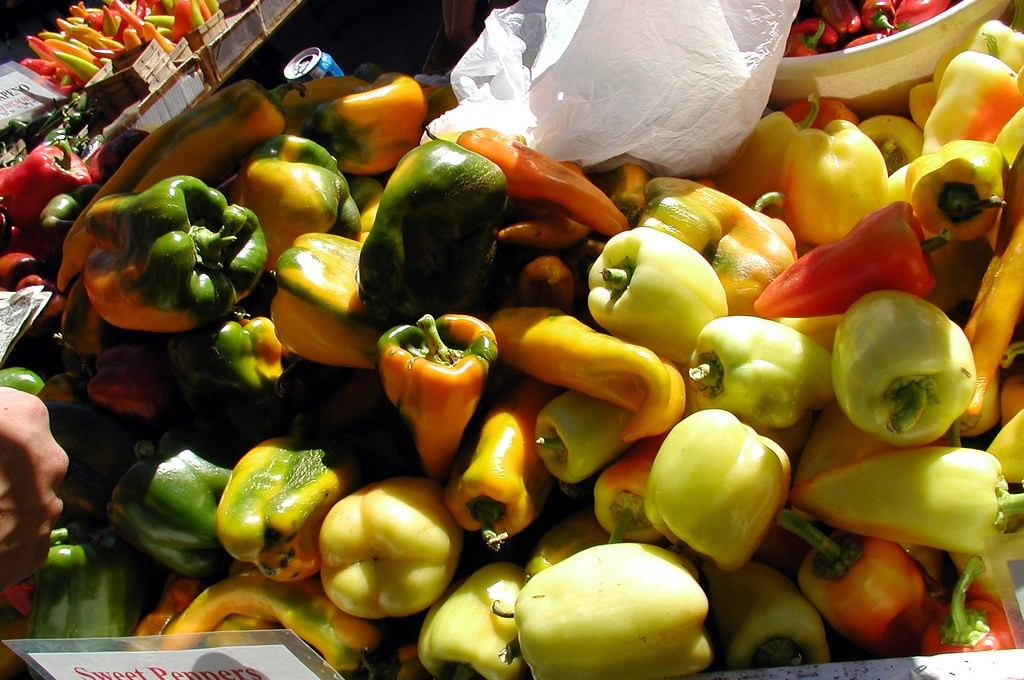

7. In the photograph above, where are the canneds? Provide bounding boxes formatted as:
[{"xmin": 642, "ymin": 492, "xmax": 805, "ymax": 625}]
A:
[{"xmin": 282, "ymin": 47, "xmax": 344, "ymax": 84}]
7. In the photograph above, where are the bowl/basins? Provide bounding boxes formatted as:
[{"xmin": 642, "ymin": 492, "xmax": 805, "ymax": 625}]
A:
[{"xmin": 766, "ymin": 0, "xmax": 1012, "ymax": 124}]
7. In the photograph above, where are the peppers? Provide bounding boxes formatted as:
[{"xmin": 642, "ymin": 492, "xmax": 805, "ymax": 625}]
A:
[{"xmin": 0, "ymin": 0, "xmax": 1024, "ymax": 680}]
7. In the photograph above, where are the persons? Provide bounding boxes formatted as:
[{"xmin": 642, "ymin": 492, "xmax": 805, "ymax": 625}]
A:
[{"xmin": 0, "ymin": 384, "xmax": 70, "ymax": 593}]
